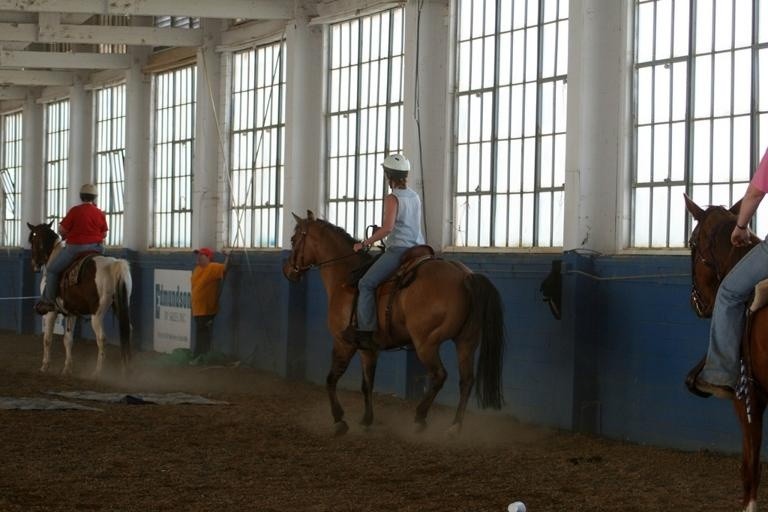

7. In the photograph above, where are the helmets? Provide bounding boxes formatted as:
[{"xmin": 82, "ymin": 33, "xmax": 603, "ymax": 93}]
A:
[{"xmin": 381, "ymin": 154, "xmax": 410, "ymax": 171}]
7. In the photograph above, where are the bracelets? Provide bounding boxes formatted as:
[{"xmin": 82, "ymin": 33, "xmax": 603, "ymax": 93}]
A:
[{"xmin": 736, "ymin": 223, "xmax": 748, "ymax": 231}]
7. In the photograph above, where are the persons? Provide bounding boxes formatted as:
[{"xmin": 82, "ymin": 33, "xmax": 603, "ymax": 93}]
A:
[
  {"xmin": 350, "ymin": 154, "xmax": 421, "ymax": 335},
  {"xmin": 32, "ymin": 184, "xmax": 108, "ymax": 311},
  {"xmin": 695, "ymin": 146, "xmax": 768, "ymax": 399},
  {"xmin": 186, "ymin": 247, "xmax": 231, "ymax": 366}
]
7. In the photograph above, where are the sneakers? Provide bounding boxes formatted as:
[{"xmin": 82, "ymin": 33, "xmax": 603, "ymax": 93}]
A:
[
  {"xmin": 189, "ymin": 354, "xmax": 207, "ymax": 365},
  {"xmin": 353, "ymin": 331, "xmax": 373, "ymax": 344},
  {"xmin": 38, "ymin": 303, "xmax": 54, "ymax": 312},
  {"xmin": 695, "ymin": 376, "xmax": 733, "ymax": 398}
]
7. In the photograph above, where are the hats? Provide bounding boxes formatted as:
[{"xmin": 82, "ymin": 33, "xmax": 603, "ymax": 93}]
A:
[
  {"xmin": 80, "ymin": 184, "xmax": 98, "ymax": 195},
  {"xmin": 193, "ymin": 248, "xmax": 213, "ymax": 259}
]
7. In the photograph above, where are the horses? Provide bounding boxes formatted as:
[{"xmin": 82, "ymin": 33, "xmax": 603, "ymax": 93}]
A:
[
  {"xmin": 26, "ymin": 218, "xmax": 133, "ymax": 379},
  {"xmin": 682, "ymin": 192, "xmax": 768, "ymax": 512},
  {"xmin": 282, "ymin": 209, "xmax": 503, "ymax": 437}
]
